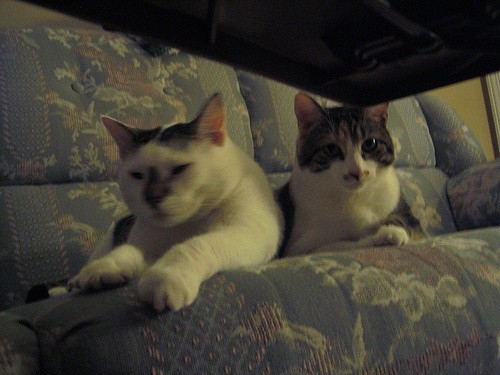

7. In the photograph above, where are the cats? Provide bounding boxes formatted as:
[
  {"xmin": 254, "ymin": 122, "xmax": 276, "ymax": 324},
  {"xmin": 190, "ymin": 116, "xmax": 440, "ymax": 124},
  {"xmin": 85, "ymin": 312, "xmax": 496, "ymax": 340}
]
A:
[
  {"xmin": 25, "ymin": 90, "xmax": 286, "ymax": 313},
  {"xmin": 271, "ymin": 93, "xmax": 427, "ymax": 261}
]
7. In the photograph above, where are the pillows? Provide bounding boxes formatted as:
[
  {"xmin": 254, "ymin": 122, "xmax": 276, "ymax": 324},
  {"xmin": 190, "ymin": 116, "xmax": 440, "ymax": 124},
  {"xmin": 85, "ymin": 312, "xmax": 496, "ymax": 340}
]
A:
[{"xmin": 447, "ymin": 161, "xmax": 500, "ymax": 232}]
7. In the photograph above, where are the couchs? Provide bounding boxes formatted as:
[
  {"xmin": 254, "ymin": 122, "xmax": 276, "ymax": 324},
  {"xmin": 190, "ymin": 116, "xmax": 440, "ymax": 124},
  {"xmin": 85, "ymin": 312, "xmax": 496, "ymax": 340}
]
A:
[{"xmin": 0, "ymin": 0, "xmax": 500, "ymax": 375}]
